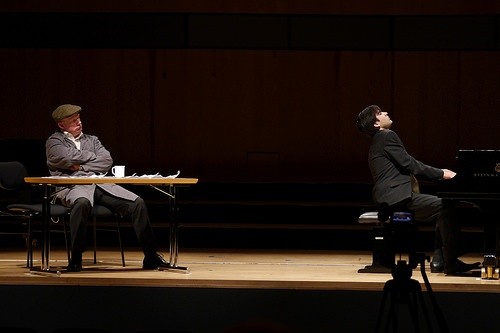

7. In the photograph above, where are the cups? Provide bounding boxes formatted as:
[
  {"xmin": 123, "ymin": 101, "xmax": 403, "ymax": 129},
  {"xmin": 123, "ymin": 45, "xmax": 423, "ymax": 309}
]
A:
[{"xmin": 111, "ymin": 165, "xmax": 125, "ymax": 178}]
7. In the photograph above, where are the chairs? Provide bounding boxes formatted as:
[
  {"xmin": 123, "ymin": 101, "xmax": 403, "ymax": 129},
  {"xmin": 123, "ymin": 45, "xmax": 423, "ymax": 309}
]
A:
[{"xmin": 0, "ymin": 160, "xmax": 126, "ymax": 271}]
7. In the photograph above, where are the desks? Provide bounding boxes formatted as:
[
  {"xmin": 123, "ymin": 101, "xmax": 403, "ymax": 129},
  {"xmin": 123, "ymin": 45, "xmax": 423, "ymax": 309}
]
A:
[{"xmin": 23, "ymin": 174, "xmax": 198, "ymax": 270}]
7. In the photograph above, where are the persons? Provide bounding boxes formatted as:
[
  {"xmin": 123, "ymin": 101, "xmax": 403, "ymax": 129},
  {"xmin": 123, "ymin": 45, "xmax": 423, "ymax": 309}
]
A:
[
  {"xmin": 356, "ymin": 105, "xmax": 481, "ymax": 272},
  {"xmin": 46, "ymin": 105, "xmax": 169, "ymax": 272}
]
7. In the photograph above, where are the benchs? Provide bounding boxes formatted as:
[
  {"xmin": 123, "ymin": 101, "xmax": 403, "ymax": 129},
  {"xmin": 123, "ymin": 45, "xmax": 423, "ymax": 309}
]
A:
[{"xmin": 358, "ymin": 211, "xmax": 410, "ymax": 273}]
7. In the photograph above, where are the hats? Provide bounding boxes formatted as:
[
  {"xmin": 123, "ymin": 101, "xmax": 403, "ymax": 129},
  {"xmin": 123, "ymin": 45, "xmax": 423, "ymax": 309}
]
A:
[{"xmin": 52, "ymin": 104, "xmax": 81, "ymax": 120}]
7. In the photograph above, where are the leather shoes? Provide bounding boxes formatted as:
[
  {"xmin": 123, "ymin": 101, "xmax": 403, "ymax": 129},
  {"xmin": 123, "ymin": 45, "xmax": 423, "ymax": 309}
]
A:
[
  {"xmin": 142, "ymin": 256, "xmax": 170, "ymax": 269},
  {"xmin": 444, "ymin": 258, "xmax": 481, "ymax": 273},
  {"xmin": 67, "ymin": 257, "xmax": 83, "ymax": 272},
  {"xmin": 430, "ymin": 260, "xmax": 444, "ymax": 273}
]
